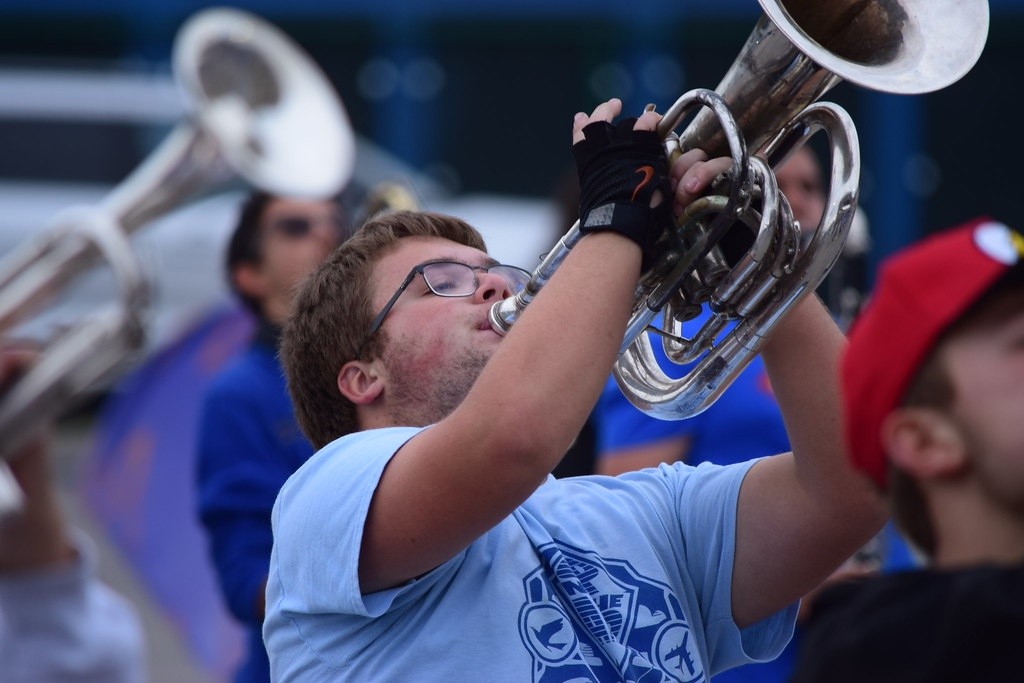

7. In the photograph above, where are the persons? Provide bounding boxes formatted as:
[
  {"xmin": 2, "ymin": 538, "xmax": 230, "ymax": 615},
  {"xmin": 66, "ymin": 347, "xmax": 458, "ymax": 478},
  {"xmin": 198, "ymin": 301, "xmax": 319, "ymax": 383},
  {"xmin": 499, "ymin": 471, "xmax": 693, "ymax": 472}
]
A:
[
  {"xmin": 793, "ymin": 221, "xmax": 1024, "ymax": 682},
  {"xmin": 262, "ymin": 99, "xmax": 891, "ymax": 683},
  {"xmin": 0, "ymin": 130, "xmax": 920, "ymax": 683}
]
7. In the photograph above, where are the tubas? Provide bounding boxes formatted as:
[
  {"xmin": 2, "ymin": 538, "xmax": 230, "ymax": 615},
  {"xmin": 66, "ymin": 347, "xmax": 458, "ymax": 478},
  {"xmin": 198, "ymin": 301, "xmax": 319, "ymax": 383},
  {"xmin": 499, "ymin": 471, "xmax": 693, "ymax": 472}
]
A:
[
  {"xmin": 486, "ymin": 1, "xmax": 993, "ymax": 423},
  {"xmin": 0, "ymin": 8, "xmax": 357, "ymax": 460}
]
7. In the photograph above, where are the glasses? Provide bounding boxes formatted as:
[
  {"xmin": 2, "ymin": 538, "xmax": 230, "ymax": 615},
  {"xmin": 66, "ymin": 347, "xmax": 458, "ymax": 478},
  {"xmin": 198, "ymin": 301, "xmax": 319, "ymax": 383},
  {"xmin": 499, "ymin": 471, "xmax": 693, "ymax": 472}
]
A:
[
  {"xmin": 256, "ymin": 213, "xmax": 341, "ymax": 265},
  {"xmin": 336, "ymin": 261, "xmax": 533, "ymax": 406}
]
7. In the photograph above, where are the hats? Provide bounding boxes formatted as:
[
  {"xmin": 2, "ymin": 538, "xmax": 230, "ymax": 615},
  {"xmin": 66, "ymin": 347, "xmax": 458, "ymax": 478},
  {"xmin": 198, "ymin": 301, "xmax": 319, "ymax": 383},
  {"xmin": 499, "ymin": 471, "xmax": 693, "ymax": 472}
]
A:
[{"xmin": 841, "ymin": 216, "xmax": 1024, "ymax": 496}]
